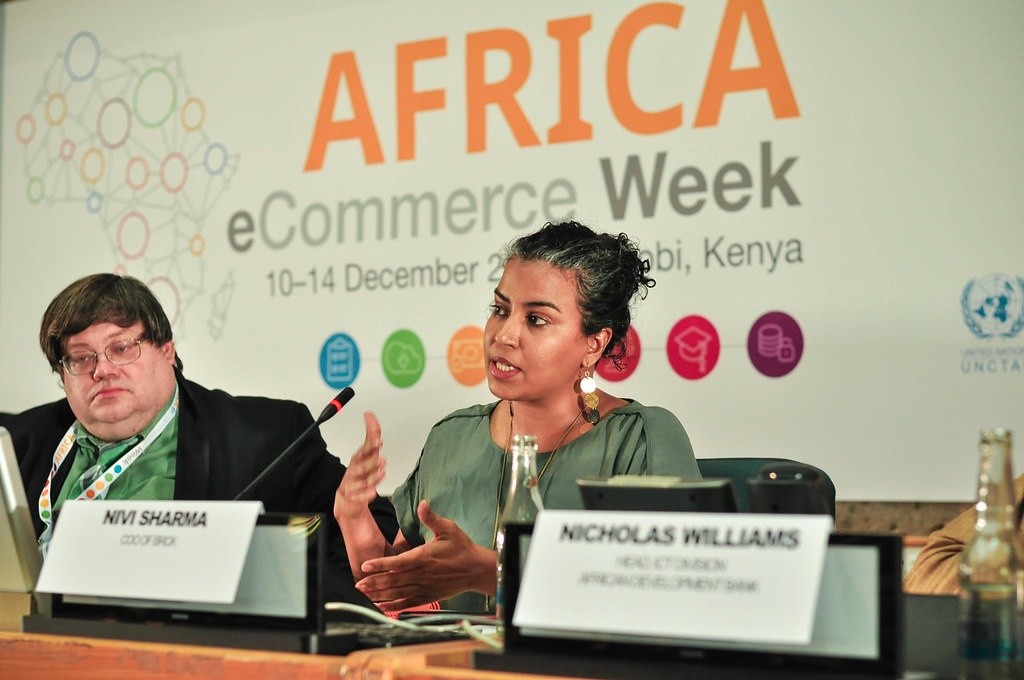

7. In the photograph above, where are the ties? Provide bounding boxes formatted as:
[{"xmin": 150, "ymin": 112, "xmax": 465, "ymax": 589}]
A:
[{"xmin": 67, "ymin": 435, "xmax": 138, "ymax": 501}]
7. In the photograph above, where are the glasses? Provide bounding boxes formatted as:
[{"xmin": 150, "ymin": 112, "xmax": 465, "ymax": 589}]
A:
[{"xmin": 59, "ymin": 337, "xmax": 149, "ymax": 375}]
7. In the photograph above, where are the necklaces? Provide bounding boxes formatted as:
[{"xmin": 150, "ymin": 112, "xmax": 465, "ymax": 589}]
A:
[{"xmin": 484, "ymin": 400, "xmax": 588, "ymax": 622}]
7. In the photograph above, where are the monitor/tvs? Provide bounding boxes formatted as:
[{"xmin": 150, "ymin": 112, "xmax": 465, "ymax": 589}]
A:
[{"xmin": 576, "ymin": 473, "xmax": 739, "ymax": 512}]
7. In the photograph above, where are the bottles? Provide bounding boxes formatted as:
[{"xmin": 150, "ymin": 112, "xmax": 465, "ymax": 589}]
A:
[
  {"xmin": 495, "ymin": 435, "xmax": 545, "ymax": 637},
  {"xmin": 957, "ymin": 429, "xmax": 1024, "ymax": 680}
]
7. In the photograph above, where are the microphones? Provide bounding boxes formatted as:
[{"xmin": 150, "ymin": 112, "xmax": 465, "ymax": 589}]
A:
[{"xmin": 233, "ymin": 387, "xmax": 354, "ymax": 501}]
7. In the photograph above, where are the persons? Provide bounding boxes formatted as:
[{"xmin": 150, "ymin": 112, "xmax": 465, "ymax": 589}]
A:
[
  {"xmin": 0, "ymin": 272, "xmax": 400, "ymax": 621},
  {"xmin": 333, "ymin": 215, "xmax": 705, "ymax": 613}
]
